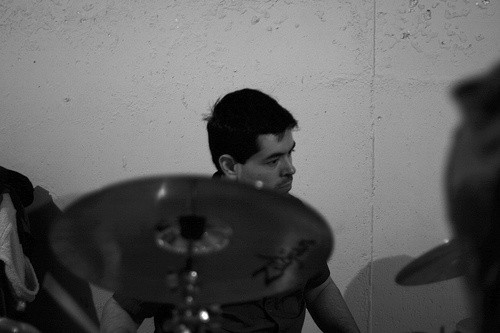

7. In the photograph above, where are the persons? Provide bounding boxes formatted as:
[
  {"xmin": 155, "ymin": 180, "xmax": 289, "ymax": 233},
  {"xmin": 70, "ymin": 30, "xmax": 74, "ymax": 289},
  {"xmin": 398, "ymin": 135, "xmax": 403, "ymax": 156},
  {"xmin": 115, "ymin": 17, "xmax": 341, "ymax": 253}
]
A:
[
  {"xmin": 0, "ymin": 164, "xmax": 44, "ymax": 333},
  {"xmin": 99, "ymin": 87, "xmax": 361, "ymax": 333}
]
